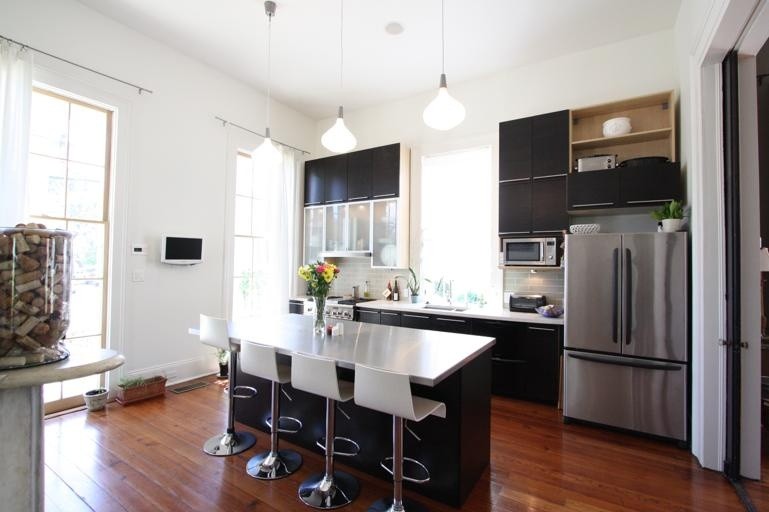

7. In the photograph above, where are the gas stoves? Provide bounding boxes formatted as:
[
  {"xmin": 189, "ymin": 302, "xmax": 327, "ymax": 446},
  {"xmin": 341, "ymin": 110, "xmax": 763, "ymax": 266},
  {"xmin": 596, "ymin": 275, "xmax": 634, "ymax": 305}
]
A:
[{"xmin": 303, "ymin": 297, "xmax": 376, "ymax": 320}]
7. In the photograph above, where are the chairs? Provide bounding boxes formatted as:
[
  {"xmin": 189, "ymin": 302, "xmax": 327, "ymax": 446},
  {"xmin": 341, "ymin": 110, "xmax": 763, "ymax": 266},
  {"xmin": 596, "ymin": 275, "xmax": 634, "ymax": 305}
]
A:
[
  {"xmin": 352, "ymin": 362, "xmax": 446, "ymax": 512},
  {"xmin": 290, "ymin": 349, "xmax": 359, "ymax": 508},
  {"xmin": 199, "ymin": 314, "xmax": 259, "ymax": 457},
  {"xmin": 239, "ymin": 340, "xmax": 303, "ymax": 479}
]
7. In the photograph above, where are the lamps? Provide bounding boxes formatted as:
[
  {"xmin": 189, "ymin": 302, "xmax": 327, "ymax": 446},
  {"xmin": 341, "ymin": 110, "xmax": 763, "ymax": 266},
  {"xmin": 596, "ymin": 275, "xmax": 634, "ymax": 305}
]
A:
[
  {"xmin": 254, "ymin": 0, "xmax": 286, "ymax": 172},
  {"xmin": 320, "ymin": 0, "xmax": 356, "ymax": 155},
  {"xmin": 422, "ymin": 1, "xmax": 466, "ymax": 131}
]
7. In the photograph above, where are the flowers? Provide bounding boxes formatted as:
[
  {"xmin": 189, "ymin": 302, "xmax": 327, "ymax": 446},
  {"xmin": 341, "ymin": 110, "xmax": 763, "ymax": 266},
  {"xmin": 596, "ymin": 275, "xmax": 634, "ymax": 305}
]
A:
[{"xmin": 299, "ymin": 260, "xmax": 340, "ymax": 317}]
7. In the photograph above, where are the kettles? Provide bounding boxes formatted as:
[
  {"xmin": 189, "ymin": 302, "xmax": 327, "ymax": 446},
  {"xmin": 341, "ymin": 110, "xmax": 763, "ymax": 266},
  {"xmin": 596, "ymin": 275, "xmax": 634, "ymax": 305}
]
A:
[{"xmin": 352, "ymin": 285, "xmax": 360, "ymax": 302}]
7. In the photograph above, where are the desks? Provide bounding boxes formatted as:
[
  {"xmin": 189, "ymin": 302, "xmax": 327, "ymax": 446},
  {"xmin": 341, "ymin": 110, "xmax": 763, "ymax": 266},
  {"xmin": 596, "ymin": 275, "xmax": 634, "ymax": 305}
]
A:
[{"xmin": 0, "ymin": 346, "xmax": 125, "ymax": 512}]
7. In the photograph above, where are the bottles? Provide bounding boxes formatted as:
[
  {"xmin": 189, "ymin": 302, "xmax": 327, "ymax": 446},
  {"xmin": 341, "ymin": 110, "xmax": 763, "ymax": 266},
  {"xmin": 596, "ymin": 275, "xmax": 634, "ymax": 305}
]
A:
[
  {"xmin": 393, "ymin": 279, "xmax": 399, "ymax": 301},
  {"xmin": 364, "ymin": 280, "xmax": 368, "ymax": 297},
  {"xmin": 386, "ymin": 280, "xmax": 392, "ymax": 300}
]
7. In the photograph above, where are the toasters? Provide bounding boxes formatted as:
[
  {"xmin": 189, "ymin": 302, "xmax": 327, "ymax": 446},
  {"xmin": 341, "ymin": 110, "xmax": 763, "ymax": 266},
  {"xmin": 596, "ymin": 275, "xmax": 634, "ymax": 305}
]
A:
[{"xmin": 509, "ymin": 291, "xmax": 547, "ymax": 313}]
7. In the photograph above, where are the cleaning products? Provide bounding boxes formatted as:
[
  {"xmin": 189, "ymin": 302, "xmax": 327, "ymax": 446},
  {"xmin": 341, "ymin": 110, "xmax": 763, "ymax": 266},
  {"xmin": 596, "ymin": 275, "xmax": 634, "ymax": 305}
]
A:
[{"xmin": 364, "ymin": 281, "xmax": 369, "ymax": 297}]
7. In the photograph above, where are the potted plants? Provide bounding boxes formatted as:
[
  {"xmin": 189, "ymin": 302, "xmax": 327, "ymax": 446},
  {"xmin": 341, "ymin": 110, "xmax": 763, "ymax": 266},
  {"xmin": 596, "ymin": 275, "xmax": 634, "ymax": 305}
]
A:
[
  {"xmin": 654, "ymin": 198, "xmax": 689, "ymax": 233},
  {"xmin": 407, "ymin": 267, "xmax": 419, "ymax": 303},
  {"xmin": 115, "ymin": 376, "xmax": 167, "ymax": 406},
  {"xmin": 218, "ymin": 349, "xmax": 229, "ymax": 377}
]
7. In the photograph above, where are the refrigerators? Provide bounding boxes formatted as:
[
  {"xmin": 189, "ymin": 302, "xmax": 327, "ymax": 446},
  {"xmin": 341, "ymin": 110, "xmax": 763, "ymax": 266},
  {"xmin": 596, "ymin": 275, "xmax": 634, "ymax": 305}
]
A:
[{"xmin": 561, "ymin": 232, "xmax": 691, "ymax": 445}]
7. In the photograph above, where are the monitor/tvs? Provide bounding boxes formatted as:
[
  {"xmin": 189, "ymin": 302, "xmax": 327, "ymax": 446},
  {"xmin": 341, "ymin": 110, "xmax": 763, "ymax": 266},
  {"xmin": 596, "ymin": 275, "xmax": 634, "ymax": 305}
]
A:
[{"xmin": 161, "ymin": 235, "xmax": 204, "ymax": 264}]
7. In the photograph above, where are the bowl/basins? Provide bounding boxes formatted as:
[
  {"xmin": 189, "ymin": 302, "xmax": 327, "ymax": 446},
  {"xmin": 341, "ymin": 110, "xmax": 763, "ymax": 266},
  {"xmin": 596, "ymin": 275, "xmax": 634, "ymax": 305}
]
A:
[
  {"xmin": 535, "ymin": 305, "xmax": 565, "ymax": 317},
  {"xmin": 602, "ymin": 117, "xmax": 632, "ymax": 138}
]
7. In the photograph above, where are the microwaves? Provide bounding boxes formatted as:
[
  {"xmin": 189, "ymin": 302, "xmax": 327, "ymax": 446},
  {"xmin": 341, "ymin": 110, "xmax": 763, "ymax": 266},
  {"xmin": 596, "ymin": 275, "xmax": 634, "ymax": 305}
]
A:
[{"xmin": 503, "ymin": 238, "xmax": 557, "ymax": 266}]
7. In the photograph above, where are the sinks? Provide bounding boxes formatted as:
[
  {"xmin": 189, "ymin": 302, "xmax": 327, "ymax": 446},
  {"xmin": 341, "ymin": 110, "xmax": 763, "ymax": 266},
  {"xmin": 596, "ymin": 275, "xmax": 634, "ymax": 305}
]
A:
[{"xmin": 423, "ymin": 305, "xmax": 468, "ymax": 312}]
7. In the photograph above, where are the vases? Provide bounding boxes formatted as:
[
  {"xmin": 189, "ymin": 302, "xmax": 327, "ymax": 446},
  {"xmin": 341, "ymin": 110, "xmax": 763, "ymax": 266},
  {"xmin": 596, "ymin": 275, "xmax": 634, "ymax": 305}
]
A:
[
  {"xmin": 313, "ymin": 292, "xmax": 327, "ymax": 329},
  {"xmin": 83, "ymin": 388, "xmax": 108, "ymax": 410}
]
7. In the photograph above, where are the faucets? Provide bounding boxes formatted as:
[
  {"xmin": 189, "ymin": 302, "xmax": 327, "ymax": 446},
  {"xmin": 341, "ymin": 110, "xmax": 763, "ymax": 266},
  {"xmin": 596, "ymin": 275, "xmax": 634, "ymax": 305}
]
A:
[{"xmin": 447, "ymin": 299, "xmax": 453, "ymax": 305}]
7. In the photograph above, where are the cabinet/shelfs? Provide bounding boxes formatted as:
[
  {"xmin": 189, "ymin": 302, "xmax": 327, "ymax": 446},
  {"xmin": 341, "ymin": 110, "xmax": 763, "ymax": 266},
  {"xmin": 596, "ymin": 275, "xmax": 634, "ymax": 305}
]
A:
[
  {"xmin": 569, "ymin": 88, "xmax": 679, "ymax": 216},
  {"xmin": 371, "ymin": 198, "xmax": 410, "ymax": 270},
  {"xmin": 499, "ymin": 109, "xmax": 569, "ymax": 235},
  {"xmin": 304, "ymin": 153, "xmax": 348, "ymax": 206},
  {"xmin": 322, "ymin": 200, "xmax": 372, "ymax": 257},
  {"xmin": 303, "ymin": 205, "xmax": 324, "ymax": 266},
  {"xmin": 348, "ymin": 142, "xmax": 411, "ymax": 202}
]
